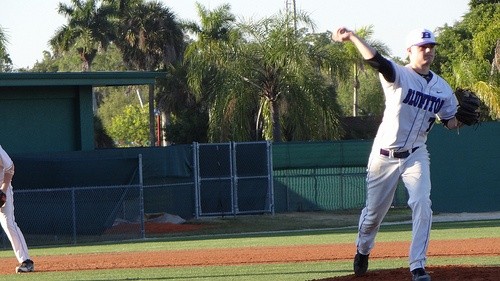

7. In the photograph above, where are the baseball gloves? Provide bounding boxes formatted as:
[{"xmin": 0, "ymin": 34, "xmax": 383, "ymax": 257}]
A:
[
  {"xmin": 453, "ymin": 86, "xmax": 484, "ymax": 128},
  {"xmin": 0, "ymin": 190, "xmax": 6, "ymax": 208}
]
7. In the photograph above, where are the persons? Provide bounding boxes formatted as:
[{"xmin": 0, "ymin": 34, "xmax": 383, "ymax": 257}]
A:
[
  {"xmin": 0, "ymin": 143, "xmax": 35, "ymax": 273},
  {"xmin": 332, "ymin": 27, "xmax": 468, "ymax": 281}
]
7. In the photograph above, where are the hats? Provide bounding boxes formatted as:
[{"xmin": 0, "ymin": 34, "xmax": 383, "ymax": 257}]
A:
[{"xmin": 406, "ymin": 27, "xmax": 441, "ymax": 47}]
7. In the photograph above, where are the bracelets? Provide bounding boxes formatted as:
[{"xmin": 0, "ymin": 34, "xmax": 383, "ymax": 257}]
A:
[{"xmin": 454, "ymin": 116, "xmax": 462, "ymax": 136}]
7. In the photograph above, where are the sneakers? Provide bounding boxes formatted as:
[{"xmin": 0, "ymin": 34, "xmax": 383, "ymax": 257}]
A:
[
  {"xmin": 354, "ymin": 244, "xmax": 370, "ymax": 277},
  {"xmin": 15, "ymin": 259, "xmax": 34, "ymax": 272},
  {"xmin": 411, "ymin": 268, "xmax": 430, "ymax": 281}
]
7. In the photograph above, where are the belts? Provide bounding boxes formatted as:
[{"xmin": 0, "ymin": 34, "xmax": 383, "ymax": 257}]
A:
[{"xmin": 381, "ymin": 145, "xmax": 419, "ymax": 159}]
